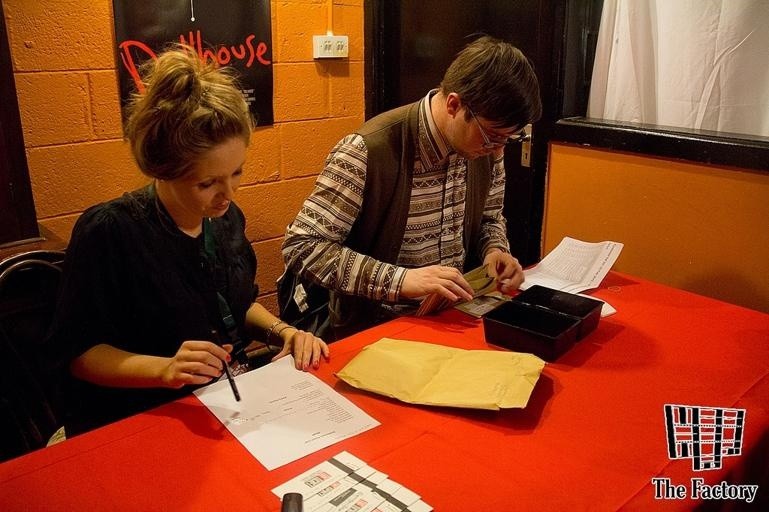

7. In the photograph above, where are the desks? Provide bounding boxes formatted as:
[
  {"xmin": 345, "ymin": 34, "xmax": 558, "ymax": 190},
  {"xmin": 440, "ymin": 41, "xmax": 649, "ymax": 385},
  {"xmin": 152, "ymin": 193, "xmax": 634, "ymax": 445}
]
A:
[{"xmin": 1, "ymin": 262, "xmax": 769, "ymax": 512}]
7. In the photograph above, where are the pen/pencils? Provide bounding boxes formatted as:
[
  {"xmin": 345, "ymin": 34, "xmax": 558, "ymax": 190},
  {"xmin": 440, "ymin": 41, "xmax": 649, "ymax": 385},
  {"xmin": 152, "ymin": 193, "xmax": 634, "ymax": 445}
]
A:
[{"xmin": 211, "ymin": 330, "xmax": 240, "ymax": 401}]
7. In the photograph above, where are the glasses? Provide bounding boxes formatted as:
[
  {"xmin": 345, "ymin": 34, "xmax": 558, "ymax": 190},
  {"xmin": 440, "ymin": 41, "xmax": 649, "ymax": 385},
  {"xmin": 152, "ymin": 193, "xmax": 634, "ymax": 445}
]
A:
[{"xmin": 461, "ymin": 97, "xmax": 532, "ymax": 150}]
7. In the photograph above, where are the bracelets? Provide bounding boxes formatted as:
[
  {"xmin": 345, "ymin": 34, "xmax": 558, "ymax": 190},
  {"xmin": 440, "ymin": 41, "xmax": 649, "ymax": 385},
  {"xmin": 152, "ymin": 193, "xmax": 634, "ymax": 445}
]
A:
[{"xmin": 263, "ymin": 320, "xmax": 297, "ymax": 354}]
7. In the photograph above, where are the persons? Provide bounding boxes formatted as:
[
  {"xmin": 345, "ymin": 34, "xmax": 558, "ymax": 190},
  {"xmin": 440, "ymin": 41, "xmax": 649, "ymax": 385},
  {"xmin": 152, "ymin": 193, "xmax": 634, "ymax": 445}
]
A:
[
  {"xmin": 42, "ymin": 40, "xmax": 331, "ymax": 441},
  {"xmin": 276, "ymin": 32, "xmax": 543, "ymax": 347}
]
